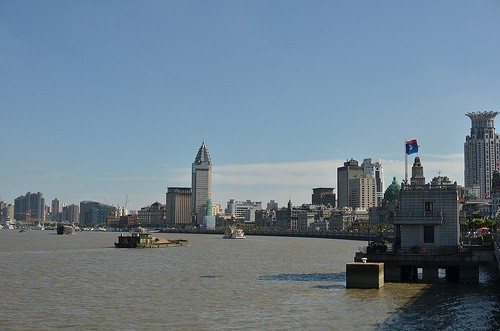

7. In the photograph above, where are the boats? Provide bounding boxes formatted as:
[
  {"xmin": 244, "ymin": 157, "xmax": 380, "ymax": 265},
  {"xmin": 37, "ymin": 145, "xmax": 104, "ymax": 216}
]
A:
[
  {"xmin": 114, "ymin": 229, "xmax": 189, "ymax": 248},
  {"xmin": 223, "ymin": 228, "xmax": 246, "ymax": 239}
]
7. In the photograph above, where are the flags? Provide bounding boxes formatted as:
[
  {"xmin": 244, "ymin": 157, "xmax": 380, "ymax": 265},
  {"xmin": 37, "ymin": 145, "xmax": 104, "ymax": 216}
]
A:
[{"xmin": 405, "ymin": 139, "xmax": 418, "ymax": 156}]
